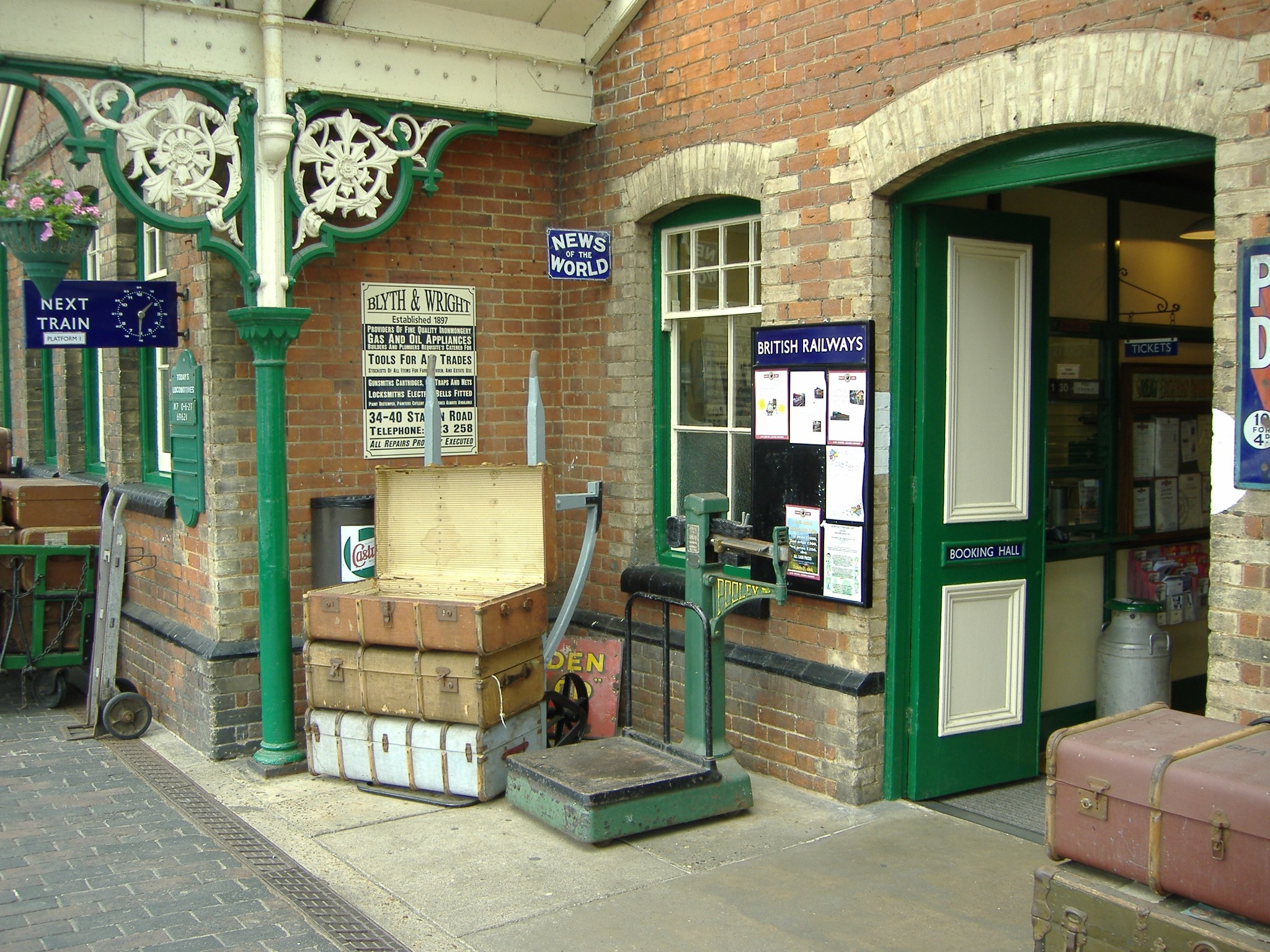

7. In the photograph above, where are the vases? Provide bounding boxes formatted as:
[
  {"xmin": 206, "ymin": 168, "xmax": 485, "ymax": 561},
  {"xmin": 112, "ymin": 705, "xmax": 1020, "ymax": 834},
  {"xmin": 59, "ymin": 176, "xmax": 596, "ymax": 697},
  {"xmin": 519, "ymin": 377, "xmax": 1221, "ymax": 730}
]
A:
[{"xmin": 0, "ymin": 217, "xmax": 99, "ymax": 301}]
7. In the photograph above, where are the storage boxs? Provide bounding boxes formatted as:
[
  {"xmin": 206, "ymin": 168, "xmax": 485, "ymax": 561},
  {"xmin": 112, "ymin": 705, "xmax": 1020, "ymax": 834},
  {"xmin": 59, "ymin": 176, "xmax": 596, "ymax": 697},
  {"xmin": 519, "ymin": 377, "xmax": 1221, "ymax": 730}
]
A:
[
  {"xmin": 302, "ymin": 460, "xmax": 558, "ymax": 803},
  {"xmin": 0, "ymin": 425, "xmax": 104, "ymax": 653},
  {"xmin": 1028, "ymin": 702, "xmax": 1270, "ymax": 952}
]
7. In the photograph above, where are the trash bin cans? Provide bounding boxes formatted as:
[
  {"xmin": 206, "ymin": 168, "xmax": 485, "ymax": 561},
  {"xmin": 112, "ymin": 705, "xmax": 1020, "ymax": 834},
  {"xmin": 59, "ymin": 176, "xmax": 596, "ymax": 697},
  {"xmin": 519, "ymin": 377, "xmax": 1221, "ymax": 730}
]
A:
[{"xmin": 308, "ymin": 492, "xmax": 375, "ymax": 588}]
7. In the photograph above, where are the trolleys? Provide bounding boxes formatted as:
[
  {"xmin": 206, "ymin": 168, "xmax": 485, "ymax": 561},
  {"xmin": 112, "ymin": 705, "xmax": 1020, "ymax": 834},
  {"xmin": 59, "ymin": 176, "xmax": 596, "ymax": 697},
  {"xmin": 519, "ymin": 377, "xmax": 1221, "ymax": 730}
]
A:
[
  {"xmin": 356, "ymin": 479, "xmax": 605, "ymax": 806},
  {"xmin": 67, "ymin": 488, "xmax": 152, "ymax": 741}
]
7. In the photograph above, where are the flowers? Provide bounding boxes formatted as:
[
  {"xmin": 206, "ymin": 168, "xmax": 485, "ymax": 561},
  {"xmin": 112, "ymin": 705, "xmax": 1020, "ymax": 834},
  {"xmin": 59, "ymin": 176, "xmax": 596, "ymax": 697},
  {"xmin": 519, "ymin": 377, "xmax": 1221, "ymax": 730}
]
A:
[{"xmin": 0, "ymin": 169, "xmax": 105, "ymax": 242}]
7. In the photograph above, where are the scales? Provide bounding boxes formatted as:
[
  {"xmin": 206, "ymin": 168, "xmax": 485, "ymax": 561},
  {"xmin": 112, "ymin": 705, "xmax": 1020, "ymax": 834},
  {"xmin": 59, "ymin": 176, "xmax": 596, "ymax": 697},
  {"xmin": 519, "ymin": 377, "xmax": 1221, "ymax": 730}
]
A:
[{"xmin": 502, "ymin": 487, "xmax": 793, "ymax": 848}]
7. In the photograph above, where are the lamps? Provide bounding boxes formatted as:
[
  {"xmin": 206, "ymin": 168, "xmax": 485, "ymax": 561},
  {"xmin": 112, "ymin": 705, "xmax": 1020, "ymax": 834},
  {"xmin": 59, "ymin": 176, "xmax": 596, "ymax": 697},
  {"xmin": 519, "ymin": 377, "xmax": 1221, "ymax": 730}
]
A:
[{"xmin": 1180, "ymin": 215, "xmax": 1215, "ymax": 241}]
